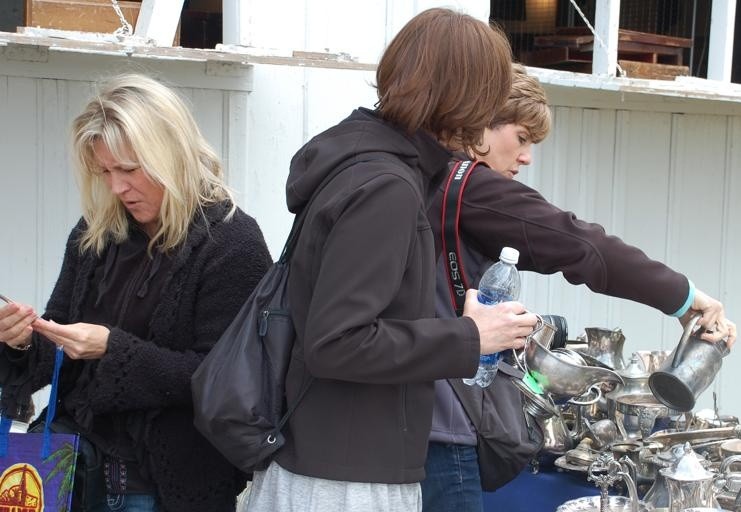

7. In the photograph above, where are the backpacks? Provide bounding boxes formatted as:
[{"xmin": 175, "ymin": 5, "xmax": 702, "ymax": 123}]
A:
[
  {"xmin": 190, "ymin": 261, "xmax": 318, "ymax": 477},
  {"xmin": 446, "ymin": 359, "xmax": 535, "ymax": 492}
]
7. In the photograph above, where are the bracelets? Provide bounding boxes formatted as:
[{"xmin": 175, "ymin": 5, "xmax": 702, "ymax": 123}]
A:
[{"xmin": 10, "ymin": 344, "xmax": 32, "ymax": 352}]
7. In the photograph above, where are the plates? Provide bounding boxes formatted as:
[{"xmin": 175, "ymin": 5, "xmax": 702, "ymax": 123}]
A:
[{"xmin": 553, "ymin": 496, "xmax": 649, "ymax": 512}]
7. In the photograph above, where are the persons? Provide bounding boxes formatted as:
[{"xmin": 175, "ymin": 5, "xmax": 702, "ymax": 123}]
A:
[
  {"xmin": 235, "ymin": 9, "xmax": 539, "ymax": 512},
  {"xmin": 0, "ymin": 72, "xmax": 273, "ymax": 512},
  {"xmin": 422, "ymin": 63, "xmax": 738, "ymax": 512}
]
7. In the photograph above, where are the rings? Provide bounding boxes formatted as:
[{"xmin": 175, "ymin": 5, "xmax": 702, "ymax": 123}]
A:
[{"xmin": 56, "ymin": 343, "xmax": 64, "ymax": 353}]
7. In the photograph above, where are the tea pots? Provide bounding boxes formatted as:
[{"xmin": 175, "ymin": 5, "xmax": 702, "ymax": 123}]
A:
[
  {"xmin": 512, "ymin": 311, "xmax": 624, "ymax": 419},
  {"xmin": 647, "ymin": 314, "xmax": 730, "ymax": 411}
]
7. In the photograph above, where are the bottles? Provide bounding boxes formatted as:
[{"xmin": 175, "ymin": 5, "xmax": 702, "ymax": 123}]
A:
[{"xmin": 462, "ymin": 246, "xmax": 521, "ymax": 388}]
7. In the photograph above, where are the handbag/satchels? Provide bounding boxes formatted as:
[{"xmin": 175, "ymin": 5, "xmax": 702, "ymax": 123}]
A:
[
  {"xmin": 191, "ymin": 159, "xmax": 428, "ymax": 473},
  {"xmin": 442, "ymin": 160, "xmax": 553, "ymax": 491},
  {"xmin": 0, "ymin": 341, "xmax": 84, "ymax": 512}
]
